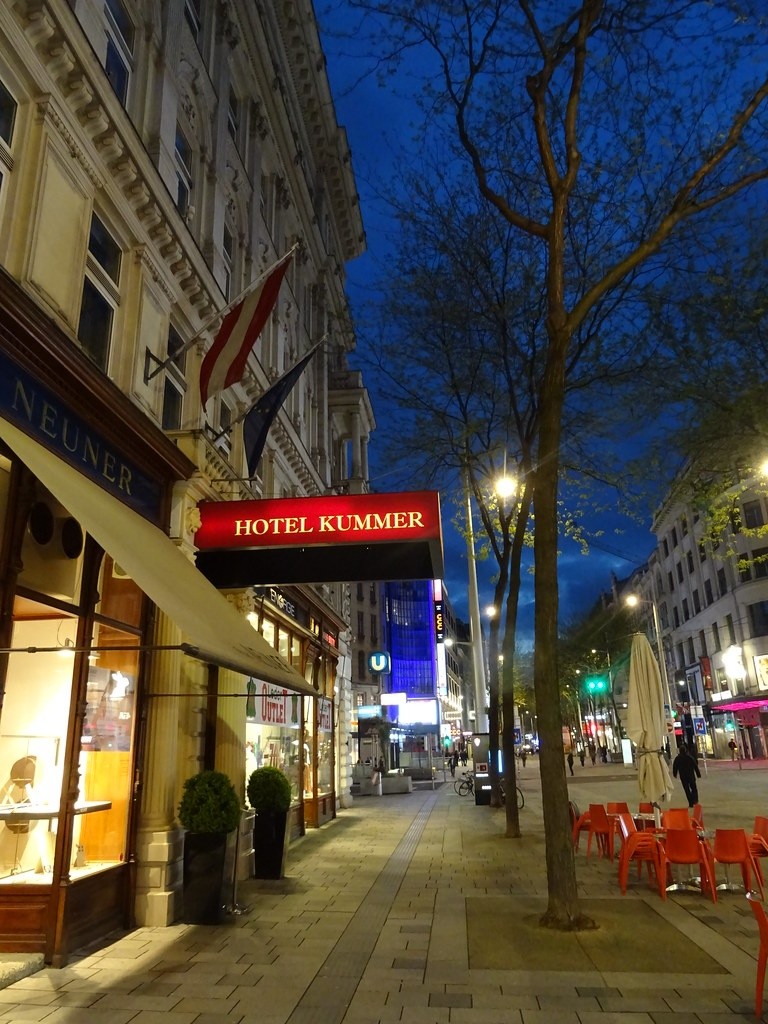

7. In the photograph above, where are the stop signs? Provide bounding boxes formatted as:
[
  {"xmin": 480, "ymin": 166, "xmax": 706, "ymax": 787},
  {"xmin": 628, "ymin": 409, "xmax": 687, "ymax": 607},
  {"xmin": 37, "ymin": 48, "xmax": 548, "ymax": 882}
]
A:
[{"xmin": 666, "ymin": 723, "xmax": 674, "ymax": 734}]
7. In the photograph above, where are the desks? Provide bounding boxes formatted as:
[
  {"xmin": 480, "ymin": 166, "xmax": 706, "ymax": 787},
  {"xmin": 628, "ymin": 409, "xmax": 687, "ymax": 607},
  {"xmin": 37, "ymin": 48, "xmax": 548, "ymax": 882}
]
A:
[
  {"xmin": 605, "ymin": 813, "xmax": 664, "ymax": 833},
  {"xmin": 654, "ymin": 830, "xmax": 746, "ymax": 894}
]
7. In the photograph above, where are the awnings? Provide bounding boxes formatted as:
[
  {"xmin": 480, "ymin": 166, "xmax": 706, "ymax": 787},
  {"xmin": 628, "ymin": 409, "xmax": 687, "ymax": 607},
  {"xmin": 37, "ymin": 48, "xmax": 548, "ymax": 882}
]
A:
[{"xmin": 0, "ymin": 417, "xmax": 322, "ymax": 697}]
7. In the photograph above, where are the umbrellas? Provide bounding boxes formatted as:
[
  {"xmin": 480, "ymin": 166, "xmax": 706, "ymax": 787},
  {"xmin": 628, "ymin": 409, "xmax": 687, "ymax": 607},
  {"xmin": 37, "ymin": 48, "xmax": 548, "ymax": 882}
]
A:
[{"xmin": 626, "ymin": 632, "xmax": 674, "ymax": 827}]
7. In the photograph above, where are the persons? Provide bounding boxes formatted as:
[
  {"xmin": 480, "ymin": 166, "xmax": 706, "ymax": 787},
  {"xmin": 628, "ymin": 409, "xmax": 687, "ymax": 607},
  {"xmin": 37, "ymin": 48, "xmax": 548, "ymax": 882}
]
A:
[
  {"xmin": 578, "ymin": 749, "xmax": 585, "ymax": 767},
  {"xmin": 672, "ymin": 745, "xmax": 701, "ymax": 808},
  {"xmin": 357, "ymin": 756, "xmax": 384, "ymax": 768},
  {"xmin": 567, "ymin": 751, "xmax": 575, "ymax": 776},
  {"xmin": 589, "ymin": 745, "xmax": 608, "ymax": 766},
  {"xmin": 444, "ymin": 746, "xmax": 469, "ymax": 777},
  {"xmin": 531, "ymin": 748, "xmax": 534, "ymax": 756},
  {"xmin": 521, "ymin": 749, "xmax": 527, "ymax": 767},
  {"xmin": 729, "ymin": 738, "xmax": 738, "ymax": 761}
]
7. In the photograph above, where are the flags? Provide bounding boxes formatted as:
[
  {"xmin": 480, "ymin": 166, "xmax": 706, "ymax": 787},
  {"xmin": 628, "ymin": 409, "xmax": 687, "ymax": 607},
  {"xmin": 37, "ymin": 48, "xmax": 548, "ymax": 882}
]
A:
[
  {"xmin": 198, "ymin": 257, "xmax": 289, "ymax": 412},
  {"xmin": 243, "ymin": 347, "xmax": 318, "ymax": 477}
]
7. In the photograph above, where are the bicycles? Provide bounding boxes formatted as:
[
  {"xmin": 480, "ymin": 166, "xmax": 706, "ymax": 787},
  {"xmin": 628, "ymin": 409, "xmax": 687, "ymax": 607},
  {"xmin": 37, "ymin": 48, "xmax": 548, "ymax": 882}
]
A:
[
  {"xmin": 454, "ymin": 770, "xmax": 474, "ymax": 797},
  {"xmin": 498, "ymin": 774, "xmax": 525, "ymax": 808}
]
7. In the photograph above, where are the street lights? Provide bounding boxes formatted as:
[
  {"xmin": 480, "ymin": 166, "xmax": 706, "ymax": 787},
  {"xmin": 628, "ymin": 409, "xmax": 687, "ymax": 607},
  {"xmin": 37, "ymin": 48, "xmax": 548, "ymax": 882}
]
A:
[
  {"xmin": 626, "ymin": 594, "xmax": 678, "ymax": 765},
  {"xmin": 592, "ymin": 649, "xmax": 618, "ymax": 726}
]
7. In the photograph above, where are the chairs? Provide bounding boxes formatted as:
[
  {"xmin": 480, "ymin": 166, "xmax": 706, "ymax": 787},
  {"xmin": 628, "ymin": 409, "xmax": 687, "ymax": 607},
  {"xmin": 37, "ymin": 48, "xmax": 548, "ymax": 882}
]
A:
[
  {"xmin": 639, "ymin": 801, "xmax": 662, "ymax": 814},
  {"xmin": 616, "ymin": 813, "xmax": 664, "ymax": 895},
  {"xmin": 706, "ymin": 830, "xmax": 765, "ymax": 902},
  {"xmin": 569, "ymin": 800, "xmax": 615, "ymax": 864},
  {"xmin": 660, "ymin": 830, "xmax": 716, "ymax": 903},
  {"xmin": 662, "ymin": 810, "xmax": 700, "ymax": 886},
  {"xmin": 607, "ymin": 802, "xmax": 629, "ymax": 814},
  {"xmin": 743, "ymin": 817, "xmax": 768, "ymax": 892}
]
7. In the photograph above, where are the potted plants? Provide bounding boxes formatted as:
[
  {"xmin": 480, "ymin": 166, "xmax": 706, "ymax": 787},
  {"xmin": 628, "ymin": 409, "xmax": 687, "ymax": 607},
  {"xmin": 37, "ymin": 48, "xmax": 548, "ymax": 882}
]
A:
[
  {"xmin": 248, "ymin": 766, "xmax": 293, "ymax": 880},
  {"xmin": 177, "ymin": 770, "xmax": 237, "ymax": 925}
]
